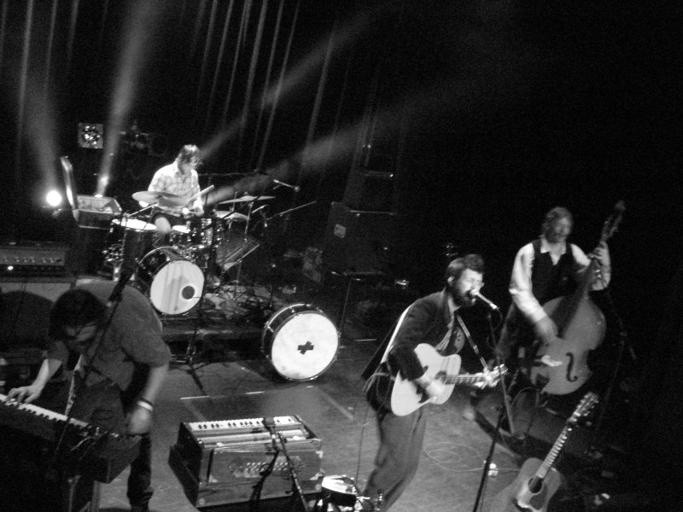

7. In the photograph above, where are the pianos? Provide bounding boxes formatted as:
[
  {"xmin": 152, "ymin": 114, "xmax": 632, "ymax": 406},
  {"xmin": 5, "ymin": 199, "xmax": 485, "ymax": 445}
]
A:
[{"xmin": 0, "ymin": 394, "xmax": 143, "ymax": 483}]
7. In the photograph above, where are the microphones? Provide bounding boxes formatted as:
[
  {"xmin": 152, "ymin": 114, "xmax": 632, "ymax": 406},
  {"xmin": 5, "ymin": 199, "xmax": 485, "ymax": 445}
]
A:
[
  {"xmin": 105, "ymin": 260, "xmax": 138, "ymax": 308},
  {"xmin": 587, "ymin": 253, "xmax": 601, "ymax": 273},
  {"xmin": 469, "ymin": 288, "xmax": 497, "ymax": 311},
  {"xmin": 263, "ymin": 417, "xmax": 277, "ymax": 440},
  {"xmin": 273, "ymin": 178, "xmax": 300, "ymax": 192}
]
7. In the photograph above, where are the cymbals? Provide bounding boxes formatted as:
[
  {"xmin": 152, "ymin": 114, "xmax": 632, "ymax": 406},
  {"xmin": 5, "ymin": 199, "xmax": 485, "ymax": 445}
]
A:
[
  {"xmin": 218, "ymin": 196, "xmax": 274, "ymax": 204},
  {"xmin": 214, "ymin": 210, "xmax": 250, "ymax": 223},
  {"xmin": 132, "ymin": 191, "xmax": 184, "ymax": 208}
]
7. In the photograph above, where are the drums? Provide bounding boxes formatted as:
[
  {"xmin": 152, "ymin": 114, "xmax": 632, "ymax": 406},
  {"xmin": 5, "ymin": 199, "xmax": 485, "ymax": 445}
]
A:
[
  {"xmin": 110, "ymin": 218, "xmax": 157, "ymax": 270},
  {"xmin": 262, "ymin": 303, "xmax": 339, "ymax": 382},
  {"xmin": 135, "ymin": 246, "xmax": 204, "ymax": 315}
]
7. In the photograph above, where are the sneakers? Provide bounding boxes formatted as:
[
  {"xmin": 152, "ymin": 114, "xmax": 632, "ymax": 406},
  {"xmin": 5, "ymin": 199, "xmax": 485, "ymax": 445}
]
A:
[
  {"xmin": 513, "ymin": 427, "xmax": 526, "ymax": 441},
  {"xmin": 463, "ymin": 401, "xmax": 476, "ymax": 421}
]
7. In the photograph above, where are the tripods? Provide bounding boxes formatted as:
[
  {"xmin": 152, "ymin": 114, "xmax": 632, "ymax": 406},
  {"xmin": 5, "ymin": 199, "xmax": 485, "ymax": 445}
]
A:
[{"xmin": 167, "ymin": 186, "xmax": 279, "ymax": 379}]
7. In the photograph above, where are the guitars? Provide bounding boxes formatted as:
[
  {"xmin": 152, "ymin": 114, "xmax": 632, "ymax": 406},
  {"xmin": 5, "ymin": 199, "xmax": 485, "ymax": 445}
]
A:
[
  {"xmin": 385, "ymin": 342, "xmax": 508, "ymax": 417},
  {"xmin": 489, "ymin": 392, "xmax": 600, "ymax": 512}
]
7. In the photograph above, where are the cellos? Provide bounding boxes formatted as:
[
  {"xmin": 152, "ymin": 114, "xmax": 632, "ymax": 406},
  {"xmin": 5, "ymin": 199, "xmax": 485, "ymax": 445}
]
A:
[{"xmin": 520, "ymin": 201, "xmax": 626, "ymax": 395}]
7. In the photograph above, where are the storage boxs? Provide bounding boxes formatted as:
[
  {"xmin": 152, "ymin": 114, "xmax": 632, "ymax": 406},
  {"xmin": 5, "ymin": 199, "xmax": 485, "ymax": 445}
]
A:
[{"xmin": 169, "ymin": 412, "xmax": 326, "ymax": 509}]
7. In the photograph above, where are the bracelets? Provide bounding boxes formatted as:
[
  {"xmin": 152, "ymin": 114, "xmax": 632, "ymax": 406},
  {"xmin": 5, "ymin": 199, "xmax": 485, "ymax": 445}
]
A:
[{"xmin": 135, "ymin": 397, "xmax": 156, "ymax": 413}]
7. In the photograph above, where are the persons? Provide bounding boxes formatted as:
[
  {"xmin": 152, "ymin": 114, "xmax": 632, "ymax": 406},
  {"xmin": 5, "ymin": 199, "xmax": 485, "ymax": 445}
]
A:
[
  {"xmin": 363, "ymin": 254, "xmax": 486, "ymax": 512},
  {"xmin": 3, "ymin": 281, "xmax": 172, "ymax": 512},
  {"xmin": 463, "ymin": 206, "xmax": 616, "ymax": 421},
  {"xmin": 147, "ymin": 143, "xmax": 205, "ymax": 244}
]
